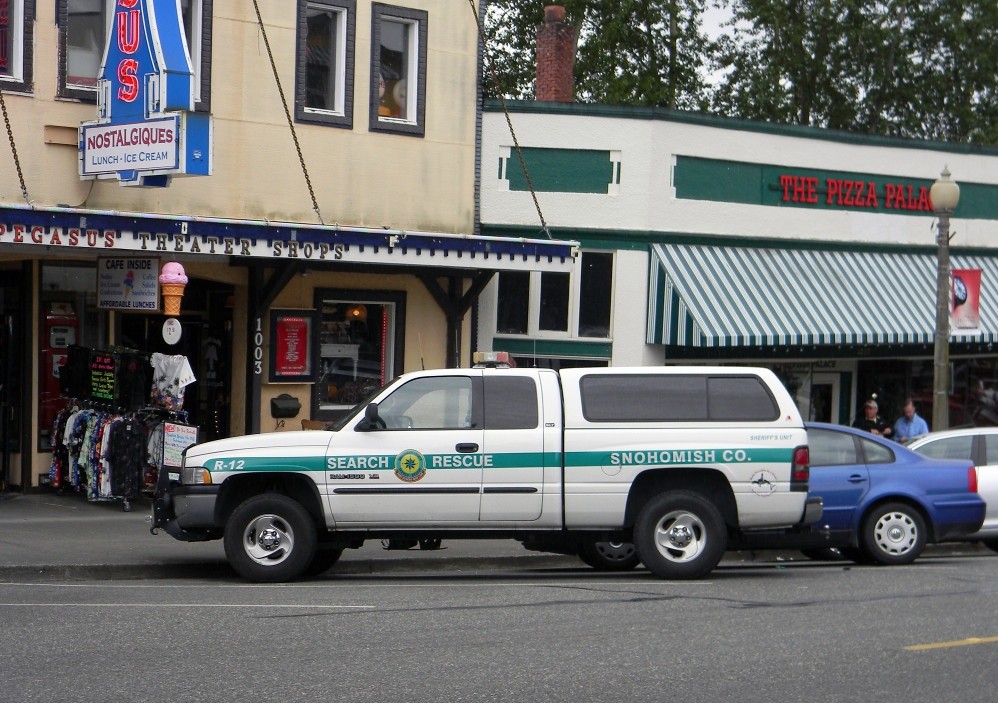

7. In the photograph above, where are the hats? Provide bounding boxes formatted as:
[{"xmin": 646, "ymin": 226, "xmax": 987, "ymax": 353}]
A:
[{"xmin": 865, "ymin": 400, "xmax": 877, "ymax": 408}]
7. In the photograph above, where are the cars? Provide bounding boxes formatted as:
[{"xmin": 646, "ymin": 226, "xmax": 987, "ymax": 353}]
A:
[
  {"xmin": 890, "ymin": 427, "xmax": 998, "ymax": 553},
  {"xmin": 730, "ymin": 419, "xmax": 986, "ymax": 566}
]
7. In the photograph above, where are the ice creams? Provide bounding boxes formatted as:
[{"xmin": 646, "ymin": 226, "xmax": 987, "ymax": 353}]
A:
[{"xmin": 158, "ymin": 262, "xmax": 188, "ymax": 316}]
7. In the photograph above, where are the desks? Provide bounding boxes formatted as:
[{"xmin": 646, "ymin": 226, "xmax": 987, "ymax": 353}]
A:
[{"xmin": 320, "ymin": 344, "xmax": 360, "ymax": 382}]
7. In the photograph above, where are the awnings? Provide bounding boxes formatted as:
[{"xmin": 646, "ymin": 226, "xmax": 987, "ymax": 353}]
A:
[{"xmin": 643, "ymin": 243, "xmax": 998, "ymax": 350}]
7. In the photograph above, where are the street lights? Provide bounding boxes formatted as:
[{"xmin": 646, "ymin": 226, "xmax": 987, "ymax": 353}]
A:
[{"xmin": 929, "ymin": 166, "xmax": 962, "ymax": 435}]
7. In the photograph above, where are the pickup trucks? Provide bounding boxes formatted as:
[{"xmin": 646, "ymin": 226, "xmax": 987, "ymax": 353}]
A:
[{"xmin": 149, "ymin": 350, "xmax": 823, "ymax": 578}]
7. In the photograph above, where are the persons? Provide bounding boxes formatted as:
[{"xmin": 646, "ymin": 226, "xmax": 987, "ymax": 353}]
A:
[
  {"xmin": 848, "ymin": 400, "xmax": 891, "ymax": 436},
  {"xmin": 893, "ymin": 400, "xmax": 929, "ymax": 443}
]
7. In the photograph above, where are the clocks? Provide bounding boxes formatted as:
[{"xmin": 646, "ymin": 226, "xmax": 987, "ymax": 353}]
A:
[{"xmin": 953, "ymin": 277, "xmax": 967, "ymax": 302}]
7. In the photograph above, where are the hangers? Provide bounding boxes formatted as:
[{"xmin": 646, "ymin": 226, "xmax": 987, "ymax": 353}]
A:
[{"xmin": 59, "ymin": 396, "xmax": 190, "ymax": 426}]
